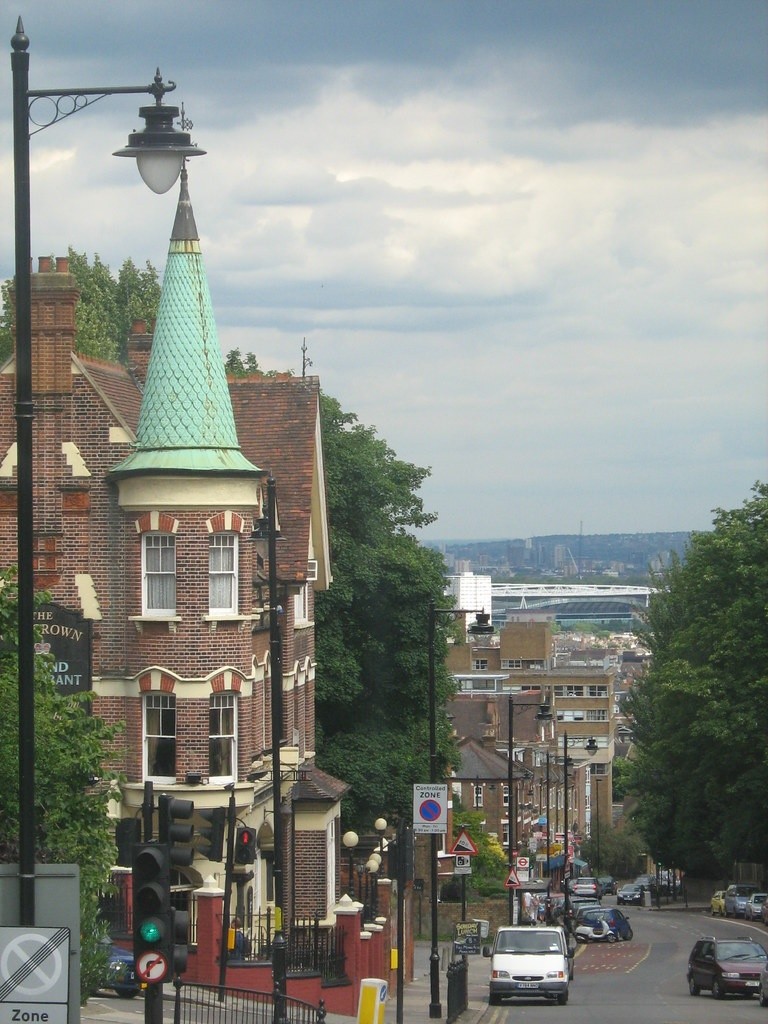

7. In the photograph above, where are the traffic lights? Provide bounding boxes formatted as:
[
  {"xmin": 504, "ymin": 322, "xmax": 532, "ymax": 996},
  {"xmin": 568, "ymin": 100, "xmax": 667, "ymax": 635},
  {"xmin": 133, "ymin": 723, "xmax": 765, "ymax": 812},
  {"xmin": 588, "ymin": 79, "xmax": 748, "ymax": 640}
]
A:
[
  {"xmin": 125, "ymin": 842, "xmax": 175, "ymax": 949},
  {"xmin": 166, "ymin": 905, "xmax": 190, "ymax": 975},
  {"xmin": 234, "ymin": 824, "xmax": 257, "ymax": 865},
  {"xmin": 198, "ymin": 805, "xmax": 226, "ymax": 863},
  {"xmin": 156, "ymin": 794, "xmax": 196, "ymax": 871}
]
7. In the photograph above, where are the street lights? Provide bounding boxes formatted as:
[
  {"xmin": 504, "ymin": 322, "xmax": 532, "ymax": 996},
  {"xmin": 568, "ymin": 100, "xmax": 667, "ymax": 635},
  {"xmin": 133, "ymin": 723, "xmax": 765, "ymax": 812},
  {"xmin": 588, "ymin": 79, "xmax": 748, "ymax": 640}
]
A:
[
  {"xmin": 366, "ymin": 854, "xmax": 383, "ymax": 917},
  {"xmin": 13, "ymin": 17, "xmax": 209, "ymax": 931},
  {"xmin": 343, "ymin": 831, "xmax": 360, "ymax": 900},
  {"xmin": 250, "ymin": 471, "xmax": 286, "ymax": 921},
  {"xmin": 365, "ymin": 860, "xmax": 378, "ymax": 923},
  {"xmin": 375, "ymin": 818, "xmax": 387, "ymax": 880},
  {"xmin": 422, "ymin": 603, "xmax": 494, "ymax": 1018},
  {"xmin": 563, "ymin": 732, "xmax": 599, "ymax": 926},
  {"xmin": 506, "ymin": 693, "xmax": 556, "ymax": 925},
  {"xmin": 545, "ymin": 751, "xmax": 574, "ymax": 876}
]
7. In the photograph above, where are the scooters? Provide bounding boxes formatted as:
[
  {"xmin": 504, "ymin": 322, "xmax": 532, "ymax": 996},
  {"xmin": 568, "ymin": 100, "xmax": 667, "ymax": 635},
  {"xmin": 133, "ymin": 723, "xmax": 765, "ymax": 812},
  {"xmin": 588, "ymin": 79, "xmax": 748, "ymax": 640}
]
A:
[{"xmin": 575, "ymin": 910, "xmax": 617, "ymax": 944}]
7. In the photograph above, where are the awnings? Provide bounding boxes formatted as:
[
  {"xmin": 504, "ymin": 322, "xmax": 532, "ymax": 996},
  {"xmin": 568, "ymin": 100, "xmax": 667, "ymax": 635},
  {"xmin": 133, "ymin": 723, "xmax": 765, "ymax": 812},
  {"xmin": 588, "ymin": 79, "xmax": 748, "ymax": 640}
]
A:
[{"xmin": 574, "ymin": 858, "xmax": 588, "ymax": 870}]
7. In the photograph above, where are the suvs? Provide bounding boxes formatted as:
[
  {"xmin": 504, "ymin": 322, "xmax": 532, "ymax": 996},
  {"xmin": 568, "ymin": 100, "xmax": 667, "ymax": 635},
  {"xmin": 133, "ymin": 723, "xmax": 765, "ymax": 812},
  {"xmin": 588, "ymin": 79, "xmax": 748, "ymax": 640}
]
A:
[
  {"xmin": 725, "ymin": 883, "xmax": 763, "ymax": 920},
  {"xmin": 686, "ymin": 935, "xmax": 768, "ymax": 1000},
  {"xmin": 483, "ymin": 925, "xmax": 574, "ymax": 1005}
]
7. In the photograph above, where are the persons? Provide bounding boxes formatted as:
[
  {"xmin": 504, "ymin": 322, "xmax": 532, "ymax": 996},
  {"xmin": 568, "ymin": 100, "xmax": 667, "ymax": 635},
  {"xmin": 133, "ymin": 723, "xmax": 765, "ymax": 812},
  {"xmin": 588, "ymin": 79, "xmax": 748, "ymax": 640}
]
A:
[{"xmin": 230, "ymin": 917, "xmax": 245, "ymax": 961}]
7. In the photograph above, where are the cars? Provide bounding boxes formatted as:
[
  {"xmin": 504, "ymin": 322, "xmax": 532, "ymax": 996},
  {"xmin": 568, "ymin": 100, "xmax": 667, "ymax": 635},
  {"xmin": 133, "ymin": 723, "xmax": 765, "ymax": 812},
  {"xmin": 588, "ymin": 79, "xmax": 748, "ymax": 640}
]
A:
[
  {"xmin": 570, "ymin": 898, "xmax": 604, "ymax": 936},
  {"xmin": 761, "ymin": 895, "xmax": 768, "ymax": 923},
  {"xmin": 744, "ymin": 893, "xmax": 768, "ymax": 921},
  {"xmin": 582, "ymin": 908, "xmax": 634, "ymax": 942},
  {"xmin": 757, "ymin": 963, "xmax": 768, "ymax": 1008},
  {"xmin": 568, "ymin": 869, "xmax": 676, "ymax": 900},
  {"xmin": 616, "ymin": 884, "xmax": 643, "ymax": 906},
  {"xmin": 79, "ymin": 925, "xmax": 143, "ymax": 1007},
  {"xmin": 709, "ymin": 891, "xmax": 727, "ymax": 918}
]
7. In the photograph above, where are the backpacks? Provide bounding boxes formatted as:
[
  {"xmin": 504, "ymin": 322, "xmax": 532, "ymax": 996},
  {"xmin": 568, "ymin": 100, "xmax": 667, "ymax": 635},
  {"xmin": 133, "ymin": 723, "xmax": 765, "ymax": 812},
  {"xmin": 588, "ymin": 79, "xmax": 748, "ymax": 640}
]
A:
[{"xmin": 236, "ymin": 931, "xmax": 252, "ymax": 959}]
